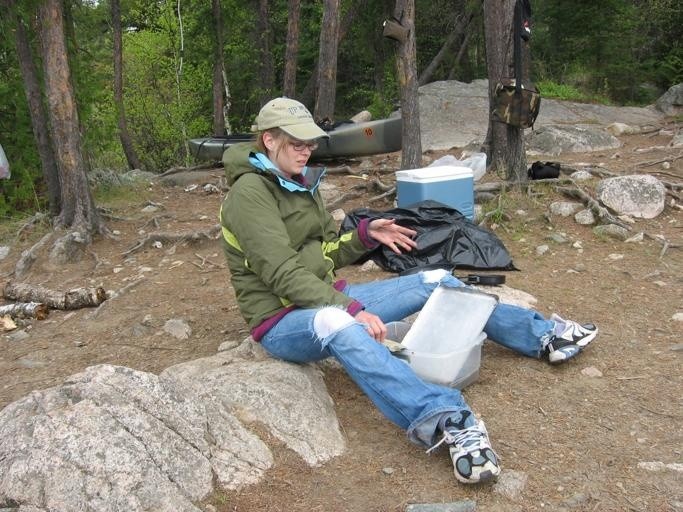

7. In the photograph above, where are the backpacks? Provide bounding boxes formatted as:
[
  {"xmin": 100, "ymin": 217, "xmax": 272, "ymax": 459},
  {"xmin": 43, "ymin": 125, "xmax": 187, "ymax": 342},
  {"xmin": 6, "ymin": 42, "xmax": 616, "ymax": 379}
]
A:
[{"xmin": 528, "ymin": 161, "xmax": 560, "ymax": 179}]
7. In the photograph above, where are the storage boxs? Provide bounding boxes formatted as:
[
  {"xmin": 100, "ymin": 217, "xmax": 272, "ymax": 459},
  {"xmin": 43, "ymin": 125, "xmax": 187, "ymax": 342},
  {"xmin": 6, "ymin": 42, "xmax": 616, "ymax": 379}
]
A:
[
  {"xmin": 395, "ymin": 165, "xmax": 474, "ymax": 217},
  {"xmin": 384, "ymin": 322, "xmax": 487, "ymax": 392}
]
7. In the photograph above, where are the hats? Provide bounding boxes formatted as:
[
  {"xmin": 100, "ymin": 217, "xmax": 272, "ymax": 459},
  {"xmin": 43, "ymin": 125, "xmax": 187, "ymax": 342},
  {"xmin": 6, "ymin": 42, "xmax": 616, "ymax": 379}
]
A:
[{"xmin": 257, "ymin": 97, "xmax": 331, "ymax": 141}]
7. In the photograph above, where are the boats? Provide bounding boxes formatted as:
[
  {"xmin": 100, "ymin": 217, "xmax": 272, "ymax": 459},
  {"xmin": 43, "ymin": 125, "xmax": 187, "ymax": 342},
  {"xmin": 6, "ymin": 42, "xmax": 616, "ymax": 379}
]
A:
[{"xmin": 185, "ymin": 113, "xmax": 403, "ymax": 168}]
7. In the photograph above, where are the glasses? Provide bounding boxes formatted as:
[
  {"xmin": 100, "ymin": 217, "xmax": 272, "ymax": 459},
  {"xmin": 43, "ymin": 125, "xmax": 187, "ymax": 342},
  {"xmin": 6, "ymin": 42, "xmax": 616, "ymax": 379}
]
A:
[{"xmin": 289, "ymin": 142, "xmax": 318, "ymax": 151}]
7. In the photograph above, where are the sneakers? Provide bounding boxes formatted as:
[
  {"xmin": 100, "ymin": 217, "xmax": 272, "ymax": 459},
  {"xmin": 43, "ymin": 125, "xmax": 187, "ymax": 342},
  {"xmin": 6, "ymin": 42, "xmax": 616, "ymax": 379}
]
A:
[
  {"xmin": 443, "ymin": 409, "xmax": 501, "ymax": 485},
  {"xmin": 545, "ymin": 313, "xmax": 599, "ymax": 365}
]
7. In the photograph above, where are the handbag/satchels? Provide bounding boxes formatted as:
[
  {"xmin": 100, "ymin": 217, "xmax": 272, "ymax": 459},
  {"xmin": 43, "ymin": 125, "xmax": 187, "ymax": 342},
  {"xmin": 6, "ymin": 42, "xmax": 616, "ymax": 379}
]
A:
[{"xmin": 490, "ymin": 80, "xmax": 541, "ymax": 129}]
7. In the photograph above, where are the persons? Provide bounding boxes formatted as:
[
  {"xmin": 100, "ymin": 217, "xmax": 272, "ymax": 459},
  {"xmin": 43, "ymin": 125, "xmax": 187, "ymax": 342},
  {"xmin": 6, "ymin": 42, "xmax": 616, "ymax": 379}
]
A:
[{"xmin": 217, "ymin": 97, "xmax": 599, "ymax": 486}]
